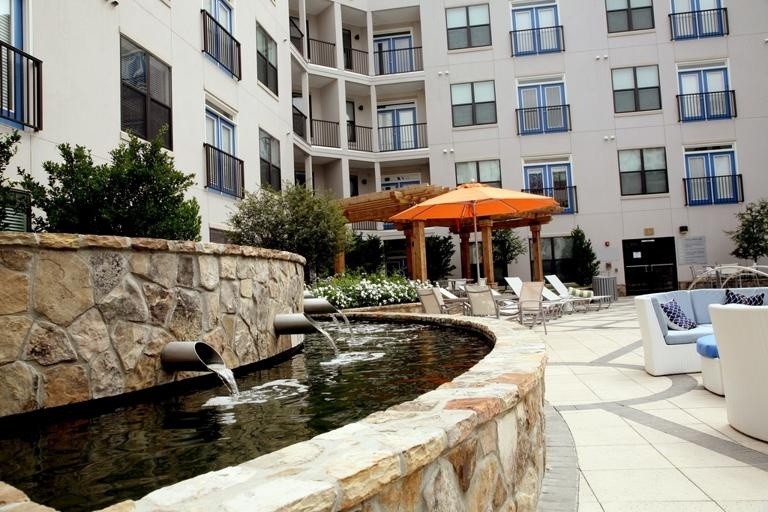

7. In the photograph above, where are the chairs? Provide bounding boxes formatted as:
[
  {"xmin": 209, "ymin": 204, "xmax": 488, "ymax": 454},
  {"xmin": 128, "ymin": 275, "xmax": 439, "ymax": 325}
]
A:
[
  {"xmin": 408, "ymin": 274, "xmax": 612, "ymax": 335},
  {"xmin": 690, "ymin": 262, "xmax": 760, "ymax": 289}
]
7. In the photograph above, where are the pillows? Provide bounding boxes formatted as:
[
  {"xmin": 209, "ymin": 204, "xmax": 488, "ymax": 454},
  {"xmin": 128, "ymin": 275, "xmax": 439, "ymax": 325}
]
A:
[{"xmin": 651, "ymin": 287, "xmax": 768, "ymax": 337}]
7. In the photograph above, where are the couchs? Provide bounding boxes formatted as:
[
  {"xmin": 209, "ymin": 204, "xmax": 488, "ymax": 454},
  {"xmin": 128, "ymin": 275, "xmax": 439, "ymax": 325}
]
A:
[{"xmin": 634, "ymin": 287, "xmax": 768, "ymax": 443}]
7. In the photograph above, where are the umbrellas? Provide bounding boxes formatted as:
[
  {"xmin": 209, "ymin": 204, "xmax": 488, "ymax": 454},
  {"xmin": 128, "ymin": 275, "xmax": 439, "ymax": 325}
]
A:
[{"xmin": 389, "ymin": 179, "xmax": 561, "ymax": 286}]
